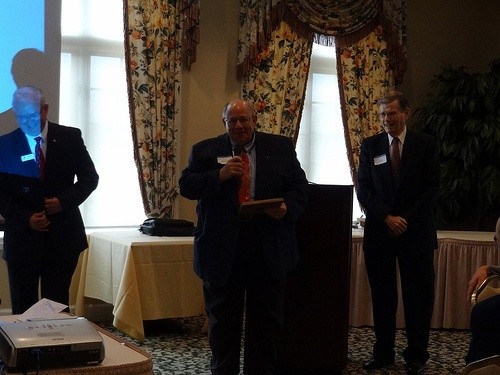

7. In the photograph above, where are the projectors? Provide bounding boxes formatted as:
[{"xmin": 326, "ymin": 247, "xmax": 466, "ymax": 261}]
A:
[{"xmin": 0, "ymin": 317, "xmax": 105, "ymax": 371}]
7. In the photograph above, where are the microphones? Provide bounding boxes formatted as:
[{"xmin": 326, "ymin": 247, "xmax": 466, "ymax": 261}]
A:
[{"xmin": 232, "ymin": 145, "xmax": 243, "ymax": 158}]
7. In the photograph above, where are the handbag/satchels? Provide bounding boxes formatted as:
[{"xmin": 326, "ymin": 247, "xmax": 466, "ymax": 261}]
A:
[{"xmin": 140, "ymin": 218, "xmax": 195, "ymax": 236}]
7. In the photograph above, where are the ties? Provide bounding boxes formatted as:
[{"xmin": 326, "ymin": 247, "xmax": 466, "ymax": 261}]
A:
[
  {"xmin": 34, "ymin": 137, "xmax": 45, "ymax": 179},
  {"xmin": 238, "ymin": 148, "xmax": 251, "ymax": 204},
  {"xmin": 392, "ymin": 137, "xmax": 400, "ymax": 175}
]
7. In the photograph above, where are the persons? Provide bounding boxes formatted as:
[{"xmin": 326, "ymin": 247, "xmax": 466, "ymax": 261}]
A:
[
  {"xmin": 357, "ymin": 91, "xmax": 439, "ymax": 375},
  {"xmin": 467, "ymin": 217, "xmax": 500, "ymax": 366},
  {"xmin": 180, "ymin": 99, "xmax": 309, "ymax": 375},
  {"xmin": 0, "ymin": 87, "xmax": 99, "ymax": 316}
]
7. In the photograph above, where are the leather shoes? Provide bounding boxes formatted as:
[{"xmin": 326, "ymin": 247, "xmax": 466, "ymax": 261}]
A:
[
  {"xmin": 362, "ymin": 359, "xmax": 394, "ymax": 370},
  {"xmin": 407, "ymin": 362, "xmax": 424, "ymax": 375}
]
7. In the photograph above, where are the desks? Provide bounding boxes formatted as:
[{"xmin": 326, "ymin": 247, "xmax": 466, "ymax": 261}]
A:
[
  {"xmin": 75, "ymin": 230, "xmax": 208, "ymax": 340},
  {"xmin": 350, "ymin": 226, "xmax": 500, "ymax": 329},
  {"xmin": 0, "ymin": 310, "xmax": 153, "ymax": 375}
]
